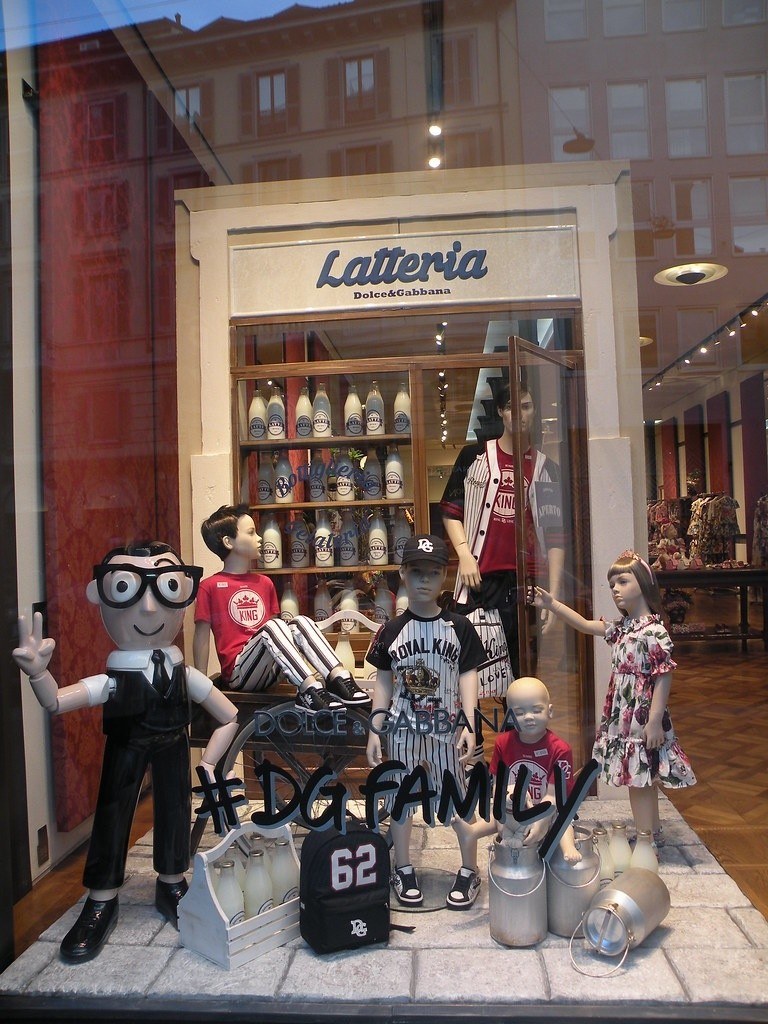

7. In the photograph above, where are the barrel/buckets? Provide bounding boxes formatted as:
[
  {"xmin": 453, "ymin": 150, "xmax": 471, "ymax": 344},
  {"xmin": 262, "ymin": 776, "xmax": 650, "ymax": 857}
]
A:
[
  {"xmin": 582, "ymin": 868, "xmax": 670, "ymax": 956},
  {"xmin": 488, "ymin": 825, "xmax": 602, "ymax": 947}
]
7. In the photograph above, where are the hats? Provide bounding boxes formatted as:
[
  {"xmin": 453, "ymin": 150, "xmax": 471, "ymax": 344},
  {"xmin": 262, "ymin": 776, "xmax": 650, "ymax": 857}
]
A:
[{"xmin": 401, "ymin": 534, "xmax": 449, "ymax": 566}]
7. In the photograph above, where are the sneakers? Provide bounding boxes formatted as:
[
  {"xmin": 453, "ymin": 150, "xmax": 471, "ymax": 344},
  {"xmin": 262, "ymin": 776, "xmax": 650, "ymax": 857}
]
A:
[
  {"xmin": 295, "ymin": 682, "xmax": 347, "ymax": 712},
  {"xmin": 446, "ymin": 866, "xmax": 481, "ymax": 907},
  {"xmin": 326, "ymin": 673, "xmax": 371, "ymax": 704},
  {"xmin": 391, "ymin": 864, "xmax": 423, "ymax": 904}
]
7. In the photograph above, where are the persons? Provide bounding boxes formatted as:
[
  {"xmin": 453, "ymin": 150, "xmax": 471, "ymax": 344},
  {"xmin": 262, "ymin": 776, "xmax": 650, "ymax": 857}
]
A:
[
  {"xmin": 438, "ymin": 381, "xmax": 566, "ymax": 783},
  {"xmin": 450, "ymin": 678, "xmax": 583, "ymax": 867},
  {"xmin": 12, "ymin": 540, "xmax": 240, "ymax": 961},
  {"xmin": 366, "ymin": 534, "xmax": 490, "ymax": 907},
  {"xmin": 659, "ymin": 523, "xmax": 688, "ymax": 570},
  {"xmin": 193, "ymin": 502, "xmax": 372, "ymax": 714},
  {"xmin": 526, "ymin": 550, "xmax": 697, "ymax": 861}
]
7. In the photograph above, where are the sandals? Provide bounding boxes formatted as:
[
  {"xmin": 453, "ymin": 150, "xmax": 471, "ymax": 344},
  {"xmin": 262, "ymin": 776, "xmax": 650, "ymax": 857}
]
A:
[
  {"xmin": 627, "ymin": 826, "xmax": 666, "ymax": 849},
  {"xmin": 651, "ymin": 841, "xmax": 660, "ymax": 861}
]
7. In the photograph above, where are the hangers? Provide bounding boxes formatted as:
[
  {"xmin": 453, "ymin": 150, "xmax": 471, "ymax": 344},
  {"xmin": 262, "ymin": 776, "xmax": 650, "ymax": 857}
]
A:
[
  {"xmin": 648, "ymin": 494, "xmax": 680, "ymax": 506},
  {"xmin": 699, "ymin": 490, "xmax": 729, "ymax": 499}
]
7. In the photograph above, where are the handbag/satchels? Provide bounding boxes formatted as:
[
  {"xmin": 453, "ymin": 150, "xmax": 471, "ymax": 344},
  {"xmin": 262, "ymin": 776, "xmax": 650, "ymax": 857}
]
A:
[
  {"xmin": 452, "ymin": 583, "xmax": 514, "ymax": 698},
  {"xmin": 664, "ymin": 556, "xmax": 703, "ymax": 570}
]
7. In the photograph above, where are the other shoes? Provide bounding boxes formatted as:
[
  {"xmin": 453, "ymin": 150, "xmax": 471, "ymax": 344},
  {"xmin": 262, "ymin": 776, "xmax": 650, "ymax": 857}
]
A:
[{"xmin": 463, "ymin": 763, "xmax": 495, "ymax": 789}]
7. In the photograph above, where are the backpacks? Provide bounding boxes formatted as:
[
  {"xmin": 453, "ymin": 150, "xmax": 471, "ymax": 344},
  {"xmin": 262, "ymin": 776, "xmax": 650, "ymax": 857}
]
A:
[{"xmin": 299, "ymin": 821, "xmax": 389, "ymax": 955}]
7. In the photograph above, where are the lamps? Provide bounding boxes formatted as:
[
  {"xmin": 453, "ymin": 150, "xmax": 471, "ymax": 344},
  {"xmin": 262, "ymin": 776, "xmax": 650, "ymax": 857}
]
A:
[
  {"xmin": 435, "ymin": 324, "xmax": 445, "ymax": 355},
  {"xmin": 646, "ymin": 304, "xmax": 764, "ymax": 390},
  {"xmin": 437, "ymin": 370, "xmax": 449, "ymax": 442},
  {"xmin": 428, "ymin": 112, "xmax": 442, "ymax": 169},
  {"xmin": 561, "ymin": 134, "xmax": 595, "ymax": 153}
]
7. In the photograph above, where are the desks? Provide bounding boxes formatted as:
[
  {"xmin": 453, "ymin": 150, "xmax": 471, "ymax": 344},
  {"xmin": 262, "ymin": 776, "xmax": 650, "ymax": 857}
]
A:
[{"xmin": 653, "ymin": 568, "xmax": 768, "ymax": 657}]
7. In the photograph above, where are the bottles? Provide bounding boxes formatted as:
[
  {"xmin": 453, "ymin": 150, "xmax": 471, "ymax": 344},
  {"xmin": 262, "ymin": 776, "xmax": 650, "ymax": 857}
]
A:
[
  {"xmin": 201, "ymin": 834, "xmax": 300, "ymax": 927},
  {"xmin": 248, "ymin": 383, "xmax": 412, "ymax": 681},
  {"xmin": 592, "ymin": 820, "xmax": 659, "ymax": 889}
]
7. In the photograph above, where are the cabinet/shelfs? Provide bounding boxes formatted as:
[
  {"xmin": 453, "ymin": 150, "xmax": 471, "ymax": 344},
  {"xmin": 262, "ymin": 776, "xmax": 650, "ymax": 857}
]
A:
[{"xmin": 225, "ymin": 368, "xmax": 424, "ymax": 675}]
